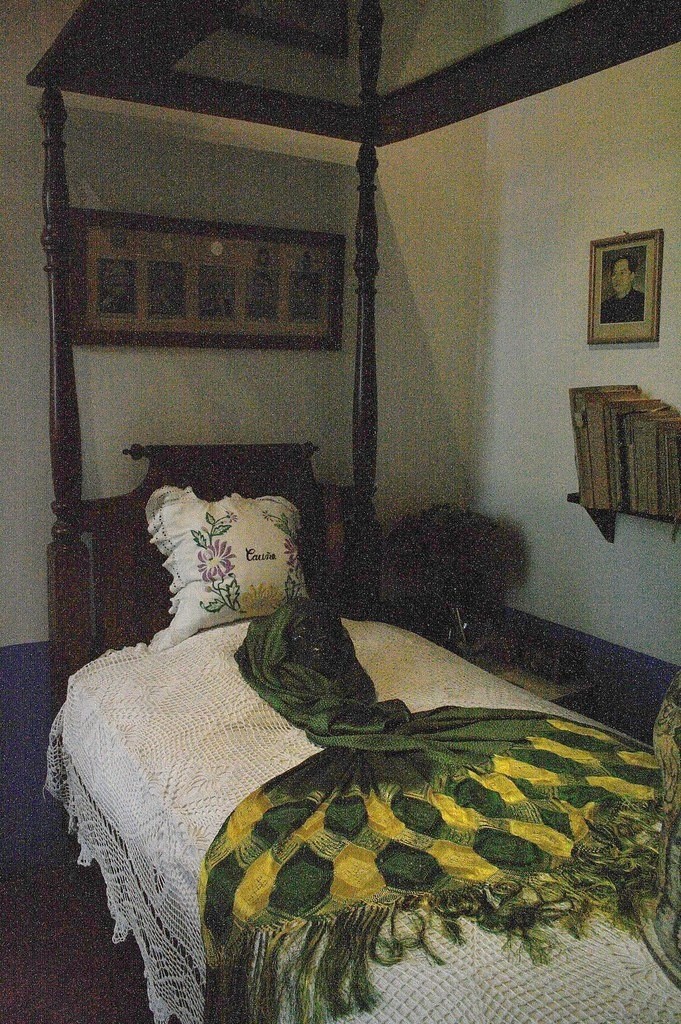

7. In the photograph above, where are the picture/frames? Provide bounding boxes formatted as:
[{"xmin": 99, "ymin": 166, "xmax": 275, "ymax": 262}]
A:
[
  {"xmin": 67, "ymin": 207, "xmax": 346, "ymax": 352},
  {"xmin": 586, "ymin": 228, "xmax": 665, "ymax": 346}
]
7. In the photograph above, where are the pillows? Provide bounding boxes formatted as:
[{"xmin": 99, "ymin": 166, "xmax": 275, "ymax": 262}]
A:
[{"xmin": 144, "ymin": 486, "xmax": 312, "ymax": 653}]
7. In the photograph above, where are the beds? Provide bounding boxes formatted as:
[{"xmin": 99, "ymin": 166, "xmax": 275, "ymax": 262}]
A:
[{"xmin": 43, "ymin": 442, "xmax": 681, "ymax": 1024}]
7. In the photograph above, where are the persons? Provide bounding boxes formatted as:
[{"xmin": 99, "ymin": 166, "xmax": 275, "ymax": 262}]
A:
[{"xmin": 601, "ymin": 254, "xmax": 645, "ymax": 323}]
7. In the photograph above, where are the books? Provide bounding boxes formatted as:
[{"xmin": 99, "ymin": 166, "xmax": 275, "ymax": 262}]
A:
[{"xmin": 569, "ymin": 385, "xmax": 681, "ymax": 517}]
